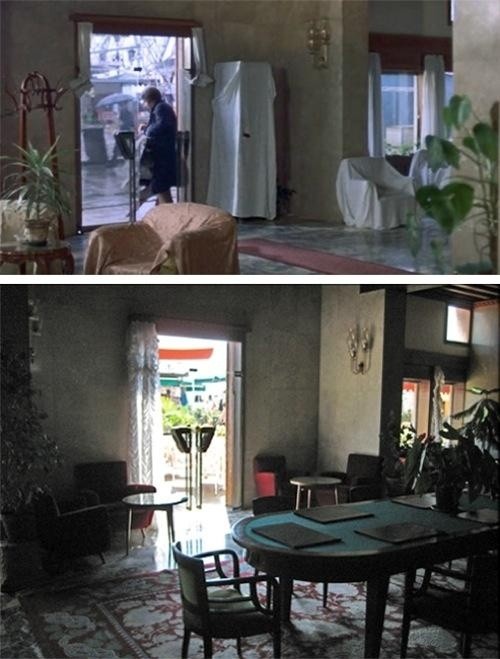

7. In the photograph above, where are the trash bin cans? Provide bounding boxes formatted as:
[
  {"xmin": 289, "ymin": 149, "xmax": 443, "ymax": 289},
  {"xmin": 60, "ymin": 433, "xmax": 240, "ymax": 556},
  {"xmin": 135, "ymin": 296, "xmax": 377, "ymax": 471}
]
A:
[
  {"xmin": 81, "ymin": 128, "xmax": 108, "ymax": 165},
  {"xmin": 253, "ymin": 454, "xmax": 288, "ymax": 497}
]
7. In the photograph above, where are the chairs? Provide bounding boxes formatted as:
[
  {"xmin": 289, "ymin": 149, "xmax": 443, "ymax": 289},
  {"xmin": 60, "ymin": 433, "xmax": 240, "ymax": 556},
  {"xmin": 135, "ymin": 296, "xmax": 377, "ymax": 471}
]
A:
[
  {"xmin": 82, "ymin": 200, "xmax": 238, "ymax": 274},
  {"xmin": 73, "ymin": 461, "xmax": 156, "ymax": 538},
  {"xmin": 0, "ymin": 198, "xmax": 64, "ymax": 274},
  {"xmin": 120, "ymin": 491, "xmax": 188, "ymax": 555},
  {"xmin": 38, "ymin": 489, "xmax": 111, "ymax": 565},
  {"xmin": 336, "ymin": 155, "xmax": 418, "ymax": 232},
  {"xmin": 400, "ymin": 550, "xmax": 500, "ymax": 659},
  {"xmin": 250, "ymin": 494, "xmax": 329, "ymax": 608},
  {"xmin": 320, "ymin": 453, "xmax": 382, "ymax": 500},
  {"xmin": 252, "ymin": 452, "xmax": 311, "ymax": 496},
  {"xmin": 171, "ymin": 542, "xmax": 281, "ymax": 658}
]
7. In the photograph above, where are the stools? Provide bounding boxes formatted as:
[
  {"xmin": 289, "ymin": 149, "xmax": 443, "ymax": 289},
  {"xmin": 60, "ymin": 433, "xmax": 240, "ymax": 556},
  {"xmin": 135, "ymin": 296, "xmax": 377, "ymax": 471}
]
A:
[{"xmin": 0, "ymin": 238, "xmax": 74, "ymax": 275}]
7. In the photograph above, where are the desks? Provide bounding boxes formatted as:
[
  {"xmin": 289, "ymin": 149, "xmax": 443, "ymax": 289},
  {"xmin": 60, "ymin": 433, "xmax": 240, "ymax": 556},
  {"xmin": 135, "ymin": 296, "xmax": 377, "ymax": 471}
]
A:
[{"xmin": 231, "ymin": 493, "xmax": 500, "ymax": 659}]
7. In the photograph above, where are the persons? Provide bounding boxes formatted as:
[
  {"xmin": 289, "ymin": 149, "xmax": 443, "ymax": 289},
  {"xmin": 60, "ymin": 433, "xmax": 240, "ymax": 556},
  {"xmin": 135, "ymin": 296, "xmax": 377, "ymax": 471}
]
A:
[{"xmin": 135, "ymin": 88, "xmax": 181, "ymax": 204}]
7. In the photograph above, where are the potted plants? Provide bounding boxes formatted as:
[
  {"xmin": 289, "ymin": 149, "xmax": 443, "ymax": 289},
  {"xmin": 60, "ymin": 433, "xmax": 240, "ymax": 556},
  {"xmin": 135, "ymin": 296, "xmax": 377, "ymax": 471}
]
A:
[
  {"xmin": 381, "ymin": 410, "xmax": 435, "ymax": 496},
  {"xmin": 1, "ymin": 303, "xmax": 64, "ymax": 544},
  {"xmin": 0, "ymin": 132, "xmax": 80, "ymax": 245},
  {"xmin": 415, "ymin": 443, "xmax": 485, "ymax": 513},
  {"xmin": 402, "ymin": 95, "xmax": 500, "ymax": 272}
]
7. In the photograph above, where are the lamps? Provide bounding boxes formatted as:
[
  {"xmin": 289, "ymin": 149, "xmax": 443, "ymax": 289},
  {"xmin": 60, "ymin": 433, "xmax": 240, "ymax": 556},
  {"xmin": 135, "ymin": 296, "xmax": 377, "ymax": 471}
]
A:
[
  {"xmin": 306, "ymin": 15, "xmax": 329, "ymax": 73},
  {"xmin": 346, "ymin": 321, "xmax": 375, "ymax": 376}
]
